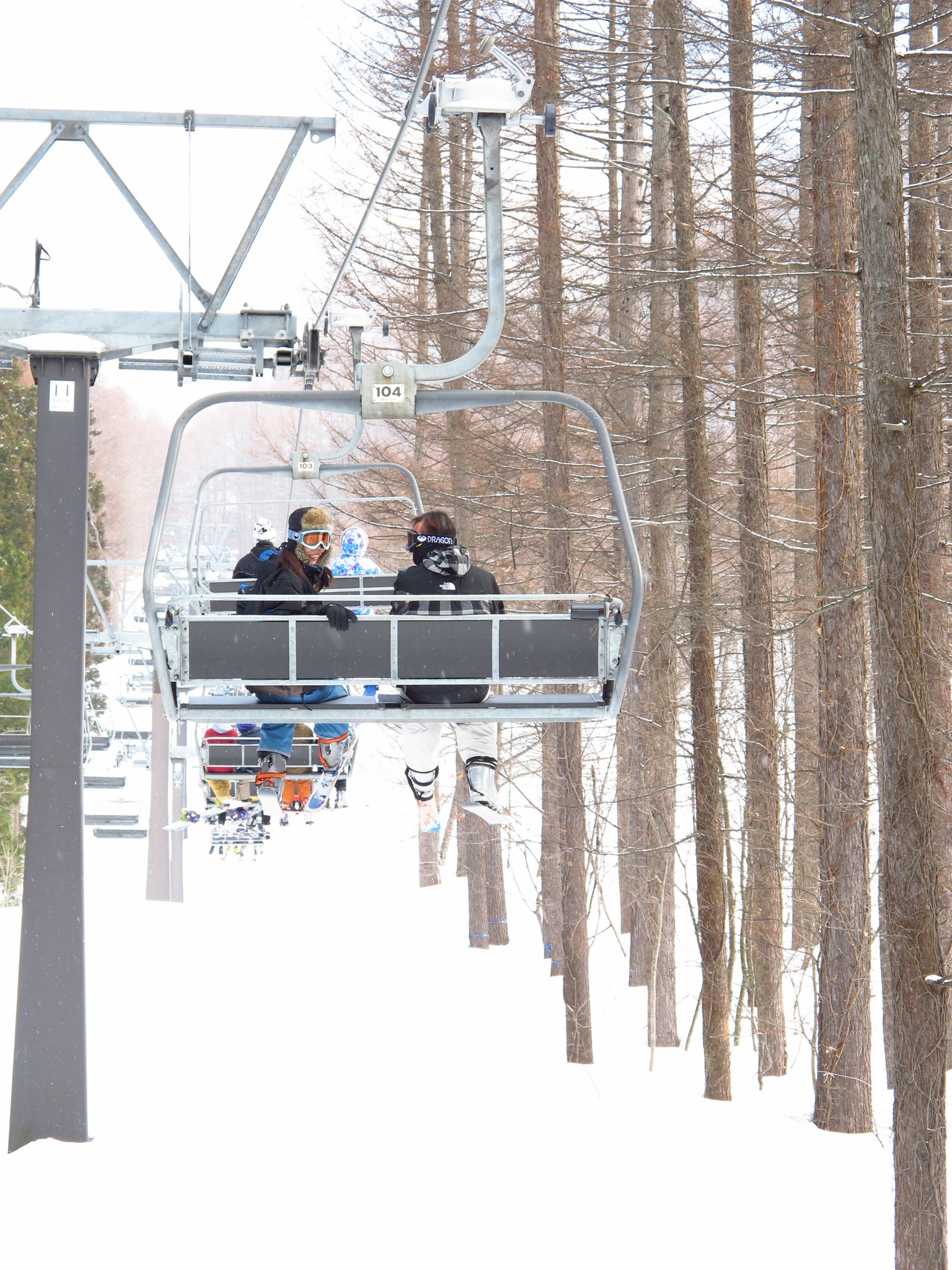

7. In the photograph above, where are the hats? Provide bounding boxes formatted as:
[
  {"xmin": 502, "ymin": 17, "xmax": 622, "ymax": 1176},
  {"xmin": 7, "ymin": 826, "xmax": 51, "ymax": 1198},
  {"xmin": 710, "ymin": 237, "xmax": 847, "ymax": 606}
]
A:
[
  {"xmin": 286, "ymin": 507, "xmax": 333, "ymax": 567},
  {"xmin": 253, "ymin": 517, "xmax": 276, "ymax": 542}
]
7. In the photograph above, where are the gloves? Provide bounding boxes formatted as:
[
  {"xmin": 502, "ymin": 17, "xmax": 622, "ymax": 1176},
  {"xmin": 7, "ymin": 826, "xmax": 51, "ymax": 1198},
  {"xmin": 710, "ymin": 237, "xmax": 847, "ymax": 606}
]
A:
[{"xmin": 318, "ymin": 603, "xmax": 358, "ymax": 631}]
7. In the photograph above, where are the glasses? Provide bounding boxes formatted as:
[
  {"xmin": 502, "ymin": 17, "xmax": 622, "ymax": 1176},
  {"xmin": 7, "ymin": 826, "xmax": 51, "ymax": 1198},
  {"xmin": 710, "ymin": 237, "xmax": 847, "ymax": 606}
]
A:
[
  {"xmin": 405, "ymin": 530, "xmax": 418, "ymax": 551},
  {"xmin": 299, "ymin": 530, "xmax": 332, "ymax": 550}
]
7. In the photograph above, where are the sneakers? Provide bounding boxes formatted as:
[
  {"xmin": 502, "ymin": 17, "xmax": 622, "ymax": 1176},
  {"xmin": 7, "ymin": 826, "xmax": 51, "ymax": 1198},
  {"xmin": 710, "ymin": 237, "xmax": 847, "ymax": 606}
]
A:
[
  {"xmin": 409, "ymin": 771, "xmax": 434, "ymax": 797},
  {"xmin": 258, "ymin": 752, "xmax": 286, "ymax": 804},
  {"xmin": 467, "ymin": 763, "xmax": 502, "ymax": 812},
  {"xmin": 319, "ymin": 727, "xmax": 355, "ymax": 766}
]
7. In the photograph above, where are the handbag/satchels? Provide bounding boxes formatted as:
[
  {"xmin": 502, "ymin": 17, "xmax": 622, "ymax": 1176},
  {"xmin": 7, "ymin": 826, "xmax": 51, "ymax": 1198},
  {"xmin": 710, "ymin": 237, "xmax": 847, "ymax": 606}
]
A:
[{"xmin": 236, "ymin": 584, "xmax": 254, "ymax": 615}]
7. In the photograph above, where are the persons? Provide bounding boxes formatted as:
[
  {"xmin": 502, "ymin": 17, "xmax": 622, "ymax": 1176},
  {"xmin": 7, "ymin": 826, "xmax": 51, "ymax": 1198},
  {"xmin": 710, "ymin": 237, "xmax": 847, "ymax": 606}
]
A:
[
  {"xmin": 324, "ymin": 528, "xmax": 386, "ymax": 696},
  {"xmin": 201, "ymin": 724, "xmax": 357, "ymax": 837},
  {"xmin": 236, "ymin": 506, "xmax": 357, "ymax": 805},
  {"xmin": 388, "ymin": 511, "xmax": 504, "ymax": 811},
  {"xmin": 232, "ymin": 516, "xmax": 285, "ymax": 615}
]
